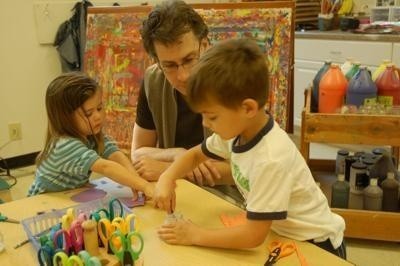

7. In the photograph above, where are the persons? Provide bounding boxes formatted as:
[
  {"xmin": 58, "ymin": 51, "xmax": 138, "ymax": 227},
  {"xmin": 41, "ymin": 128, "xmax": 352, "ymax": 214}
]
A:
[
  {"xmin": 154, "ymin": 38, "xmax": 346, "ymax": 261},
  {"xmin": 131, "ymin": 0, "xmax": 246, "ymax": 211},
  {"xmin": 27, "ymin": 71, "xmax": 154, "ymax": 201}
]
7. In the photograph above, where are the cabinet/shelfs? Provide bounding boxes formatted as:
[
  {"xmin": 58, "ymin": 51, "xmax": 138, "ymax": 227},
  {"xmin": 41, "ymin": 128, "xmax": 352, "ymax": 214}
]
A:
[
  {"xmin": 293, "ymin": 34, "xmax": 400, "ymax": 166},
  {"xmin": 300, "ymin": 86, "xmax": 400, "ymax": 244}
]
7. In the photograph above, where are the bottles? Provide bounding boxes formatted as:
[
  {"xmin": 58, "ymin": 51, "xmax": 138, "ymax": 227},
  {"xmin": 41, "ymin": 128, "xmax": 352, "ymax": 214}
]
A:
[
  {"xmin": 315, "ymin": 57, "xmax": 399, "ymax": 114},
  {"xmin": 330, "ymin": 149, "xmax": 399, "ymax": 211}
]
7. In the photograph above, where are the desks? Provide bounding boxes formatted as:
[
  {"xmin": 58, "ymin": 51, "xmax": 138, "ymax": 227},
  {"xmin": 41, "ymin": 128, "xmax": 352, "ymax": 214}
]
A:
[{"xmin": 0, "ymin": 174, "xmax": 355, "ymax": 266}]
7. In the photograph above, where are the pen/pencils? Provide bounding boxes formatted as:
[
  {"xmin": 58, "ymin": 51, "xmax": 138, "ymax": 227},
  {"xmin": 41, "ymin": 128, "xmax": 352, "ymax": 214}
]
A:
[
  {"xmin": 320, "ymin": 0, "xmax": 340, "ymax": 15},
  {"xmin": 13, "ymin": 223, "xmax": 62, "ymax": 249}
]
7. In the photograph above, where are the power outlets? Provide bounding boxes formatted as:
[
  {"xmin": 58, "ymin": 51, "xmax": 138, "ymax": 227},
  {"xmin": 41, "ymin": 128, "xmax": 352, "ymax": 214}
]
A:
[{"xmin": 7, "ymin": 121, "xmax": 24, "ymax": 142}]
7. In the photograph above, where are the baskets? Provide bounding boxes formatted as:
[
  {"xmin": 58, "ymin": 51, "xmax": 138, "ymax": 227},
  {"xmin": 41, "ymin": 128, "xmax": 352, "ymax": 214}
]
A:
[{"xmin": 22, "ymin": 194, "xmax": 136, "ymax": 262}]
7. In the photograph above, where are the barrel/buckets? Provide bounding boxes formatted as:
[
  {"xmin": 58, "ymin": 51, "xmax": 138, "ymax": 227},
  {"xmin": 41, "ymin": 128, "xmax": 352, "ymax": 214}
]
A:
[{"xmin": 312, "ymin": 59, "xmax": 400, "ymax": 114}]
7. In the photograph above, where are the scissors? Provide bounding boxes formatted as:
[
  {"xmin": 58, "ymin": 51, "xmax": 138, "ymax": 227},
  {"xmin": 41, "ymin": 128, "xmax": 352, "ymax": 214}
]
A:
[
  {"xmin": 263, "ymin": 240, "xmax": 297, "ymax": 266},
  {"xmin": 38, "ymin": 198, "xmax": 144, "ymax": 266},
  {"xmin": 0, "ymin": 212, "xmax": 20, "ymax": 224}
]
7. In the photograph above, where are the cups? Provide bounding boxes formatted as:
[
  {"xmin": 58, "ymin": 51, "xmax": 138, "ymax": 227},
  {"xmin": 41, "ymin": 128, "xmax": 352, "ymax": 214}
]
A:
[{"xmin": 318, "ymin": 14, "xmax": 335, "ymax": 32}]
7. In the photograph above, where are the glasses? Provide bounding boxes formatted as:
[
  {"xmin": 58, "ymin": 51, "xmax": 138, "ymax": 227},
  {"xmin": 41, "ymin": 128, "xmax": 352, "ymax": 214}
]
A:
[{"xmin": 155, "ymin": 39, "xmax": 201, "ymax": 73}]
